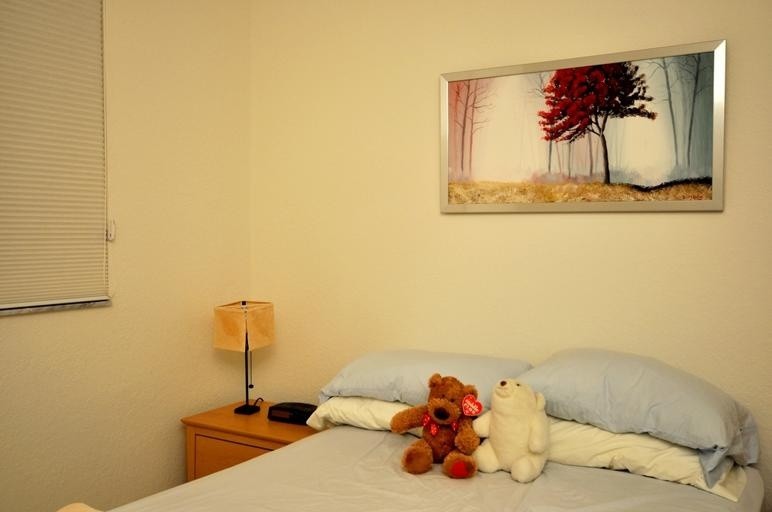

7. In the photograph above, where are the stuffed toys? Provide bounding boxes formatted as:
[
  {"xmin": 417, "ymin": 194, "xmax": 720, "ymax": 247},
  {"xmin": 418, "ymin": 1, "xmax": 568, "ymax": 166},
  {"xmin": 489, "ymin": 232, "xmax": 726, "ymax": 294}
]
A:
[
  {"xmin": 472, "ymin": 379, "xmax": 550, "ymax": 483},
  {"xmin": 389, "ymin": 373, "xmax": 480, "ymax": 480}
]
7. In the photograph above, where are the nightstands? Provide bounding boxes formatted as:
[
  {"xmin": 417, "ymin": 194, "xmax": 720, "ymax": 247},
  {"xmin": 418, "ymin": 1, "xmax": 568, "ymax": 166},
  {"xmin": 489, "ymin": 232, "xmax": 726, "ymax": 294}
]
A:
[{"xmin": 178, "ymin": 396, "xmax": 332, "ymax": 486}]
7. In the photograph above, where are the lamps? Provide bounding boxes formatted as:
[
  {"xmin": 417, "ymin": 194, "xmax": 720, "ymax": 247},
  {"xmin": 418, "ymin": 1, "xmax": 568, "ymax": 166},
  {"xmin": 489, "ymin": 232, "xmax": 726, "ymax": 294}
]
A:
[{"xmin": 212, "ymin": 299, "xmax": 281, "ymax": 415}]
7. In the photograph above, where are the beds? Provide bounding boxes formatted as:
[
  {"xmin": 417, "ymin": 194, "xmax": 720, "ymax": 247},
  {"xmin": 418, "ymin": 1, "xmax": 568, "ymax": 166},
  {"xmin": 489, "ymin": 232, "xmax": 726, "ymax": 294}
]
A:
[{"xmin": 101, "ymin": 420, "xmax": 767, "ymax": 512}]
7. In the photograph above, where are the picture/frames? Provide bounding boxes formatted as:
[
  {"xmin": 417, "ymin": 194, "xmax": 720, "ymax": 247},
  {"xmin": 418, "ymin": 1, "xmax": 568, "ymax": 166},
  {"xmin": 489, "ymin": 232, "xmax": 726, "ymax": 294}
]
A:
[{"xmin": 435, "ymin": 36, "xmax": 731, "ymax": 217}]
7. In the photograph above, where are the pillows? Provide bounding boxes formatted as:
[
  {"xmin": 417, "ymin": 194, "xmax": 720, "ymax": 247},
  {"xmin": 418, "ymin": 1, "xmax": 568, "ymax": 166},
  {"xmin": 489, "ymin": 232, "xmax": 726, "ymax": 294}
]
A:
[
  {"xmin": 543, "ymin": 414, "xmax": 750, "ymax": 503},
  {"xmin": 305, "ymin": 394, "xmax": 431, "ymax": 439},
  {"xmin": 515, "ymin": 346, "xmax": 763, "ymax": 488},
  {"xmin": 317, "ymin": 346, "xmax": 531, "ymax": 410}
]
